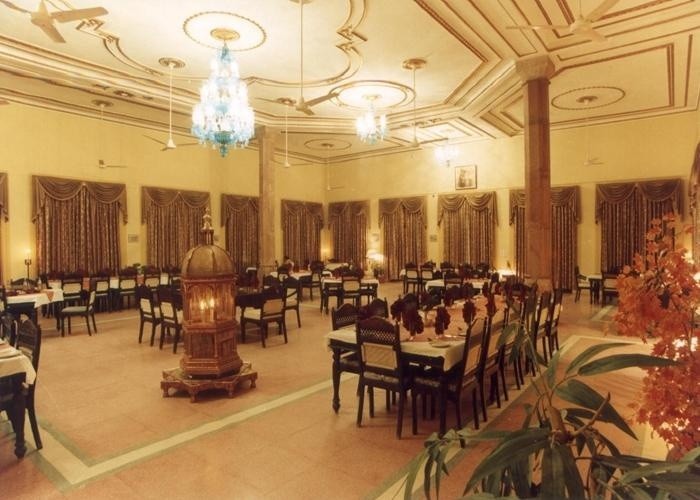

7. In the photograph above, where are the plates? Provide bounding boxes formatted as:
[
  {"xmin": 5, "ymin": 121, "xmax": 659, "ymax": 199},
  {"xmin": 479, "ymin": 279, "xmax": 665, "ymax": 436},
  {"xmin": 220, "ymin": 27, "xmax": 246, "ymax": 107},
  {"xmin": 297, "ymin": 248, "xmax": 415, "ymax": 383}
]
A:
[
  {"xmin": 0, "ymin": 349, "xmax": 23, "ymax": 358},
  {"xmin": 429, "ymin": 341, "xmax": 450, "ymax": 348}
]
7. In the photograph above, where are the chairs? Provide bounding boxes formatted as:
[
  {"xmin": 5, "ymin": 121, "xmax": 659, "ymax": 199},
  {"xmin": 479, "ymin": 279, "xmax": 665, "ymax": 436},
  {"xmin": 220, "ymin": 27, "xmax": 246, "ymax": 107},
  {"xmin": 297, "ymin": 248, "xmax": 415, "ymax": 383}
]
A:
[
  {"xmin": 0, "ymin": 320, "xmax": 42, "ymax": 450},
  {"xmin": 504, "ymin": 283, "xmax": 532, "ymax": 296},
  {"xmin": 358, "ymin": 268, "xmax": 379, "ymax": 304},
  {"xmin": 276, "ymin": 266, "xmax": 290, "ymax": 281},
  {"xmin": 136, "ymin": 284, "xmax": 171, "ymax": 347},
  {"xmin": 421, "ymin": 262, "xmax": 433, "ymax": 293},
  {"xmin": 403, "ymin": 263, "xmax": 420, "ymax": 294},
  {"xmin": 0, "ymin": 287, "xmax": 7, "ymax": 313},
  {"xmin": 279, "ymin": 276, "xmax": 301, "ymax": 329},
  {"xmin": 532, "ymin": 291, "xmax": 552, "ymax": 376},
  {"xmin": 603, "ymin": 273, "xmax": 618, "ymax": 307},
  {"xmin": 332, "ymin": 303, "xmax": 397, "ymax": 410},
  {"xmin": 318, "ymin": 270, "xmax": 340, "ymax": 312},
  {"xmin": 399, "ymin": 294, "xmax": 419, "ymax": 311},
  {"xmin": 471, "ymin": 270, "xmax": 480, "ymax": 278},
  {"xmin": 263, "ymin": 274, "xmax": 280, "ymax": 287},
  {"xmin": 120, "ymin": 267, "xmax": 136, "ymax": 310},
  {"xmin": 445, "ymin": 274, "xmax": 463, "ymax": 290},
  {"xmin": 342, "ymin": 270, "xmax": 360, "ymax": 307},
  {"xmin": 429, "ymin": 290, "xmax": 443, "ymax": 305},
  {"xmin": 479, "ymin": 310, "xmax": 506, "ymax": 422},
  {"xmin": 441, "ymin": 262, "xmax": 454, "ymax": 278},
  {"xmin": 415, "ymin": 317, "xmax": 487, "ymax": 448},
  {"xmin": 61, "ymin": 277, "xmax": 97, "ymax": 337},
  {"xmin": 94, "ymin": 270, "xmax": 109, "ymax": 312},
  {"xmin": 169, "ymin": 268, "xmax": 182, "ymax": 291},
  {"xmin": 500, "ymin": 302, "xmax": 522, "ymax": 401},
  {"xmin": 237, "ymin": 273, "xmax": 258, "ymax": 287},
  {"xmin": 44, "ymin": 272, "xmax": 62, "ymax": 318},
  {"xmin": 447, "ymin": 285, "xmax": 462, "ymax": 300},
  {"xmin": 476, "ymin": 263, "xmax": 488, "ymax": 277},
  {"xmin": 549, "ymin": 288, "xmax": 562, "ymax": 359},
  {"xmin": 63, "ymin": 273, "xmax": 82, "ymax": 307},
  {"xmin": 157, "ymin": 288, "xmax": 183, "ymax": 354},
  {"xmin": 11, "ymin": 278, "xmax": 24, "ymax": 291},
  {"xmin": 575, "ymin": 266, "xmax": 592, "ymax": 303},
  {"xmin": 473, "ymin": 288, "xmax": 480, "ymax": 295},
  {"xmin": 518, "ymin": 296, "xmax": 537, "ymax": 385},
  {"xmin": 0, "ymin": 313, "xmax": 13, "ymax": 339},
  {"xmin": 27, "ymin": 278, "xmax": 37, "ymax": 291},
  {"xmin": 146, "ymin": 267, "xmax": 160, "ymax": 289},
  {"xmin": 490, "ymin": 272, "xmax": 499, "ymax": 292},
  {"xmin": 458, "ymin": 263, "xmax": 473, "ymax": 278},
  {"xmin": 362, "ymin": 297, "xmax": 389, "ymax": 318},
  {"xmin": 243, "ymin": 284, "xmax": 288, "ymax": 348},
  {"xmin": 356, "ymin": 316, "xmax": 417, "ymax": 440},
  {"xmin": 302, "ymin": 261, "xmax": 324, "ymax": 300}
]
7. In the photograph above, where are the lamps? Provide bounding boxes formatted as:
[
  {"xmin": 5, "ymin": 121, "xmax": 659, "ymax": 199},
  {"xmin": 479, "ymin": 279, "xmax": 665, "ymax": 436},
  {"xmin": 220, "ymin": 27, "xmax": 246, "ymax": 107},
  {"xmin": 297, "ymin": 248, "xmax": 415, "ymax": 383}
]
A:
[
  {"xmin": 354, "ymin": 100, "xmax": 387, "ymax": 144},
  {"xmin": 191, "ymin": 47, "xmax": 255, "ymax": 159}
]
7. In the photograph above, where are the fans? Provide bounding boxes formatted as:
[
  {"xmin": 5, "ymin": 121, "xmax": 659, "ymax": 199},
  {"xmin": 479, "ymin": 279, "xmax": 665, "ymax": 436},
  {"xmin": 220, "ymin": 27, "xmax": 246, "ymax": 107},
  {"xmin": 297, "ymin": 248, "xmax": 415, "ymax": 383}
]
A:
[
  {"xmin": 143, "ymin": 133, "xmax": 199, "ymax": 151},
  {"xmin": 90, "ymin": 159, "xmax": 128, "ymax": 169},
  {"xmin": 255, "ymin": 93, "xmax": 339, "ymax": 115},
  {"xmin": 505, "ymin": 0, "xmax": 620, "ymax": 43},
  {"xmin": 0, "ymin": 0, "xmax": 109, "ymax": 43},
  {"xmin": 270, "ymin": 158, "xmax": 310, "ymax": 168},
  {"xmin": 391, "ymin": 136, "xmax": 448, "ymax": 150},
  {"xmin": 325, "ymin": 184, "xmax": 344, "ymax": 191},
  {"xmin": 580, "ymin": 157, "xmax": 605, "ymax": 166}
]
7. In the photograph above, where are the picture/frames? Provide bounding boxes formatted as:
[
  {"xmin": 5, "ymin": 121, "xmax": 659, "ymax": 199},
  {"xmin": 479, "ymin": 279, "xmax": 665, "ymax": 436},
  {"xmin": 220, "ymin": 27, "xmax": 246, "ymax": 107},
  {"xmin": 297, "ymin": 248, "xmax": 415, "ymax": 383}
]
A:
[{"xmin": 455, "ymin": 166, "xmax": 477, "ymax": 189}]
